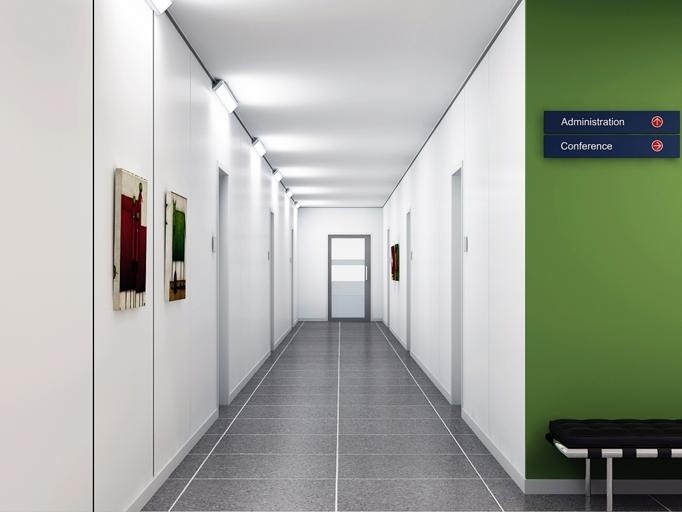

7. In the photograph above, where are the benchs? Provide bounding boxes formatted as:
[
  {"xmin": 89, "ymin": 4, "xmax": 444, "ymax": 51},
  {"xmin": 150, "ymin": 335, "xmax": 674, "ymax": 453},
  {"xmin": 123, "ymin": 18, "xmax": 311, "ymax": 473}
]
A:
[{"xmin": 543, "ymin": 420, "xmax": 682, "ymax": 512}]
